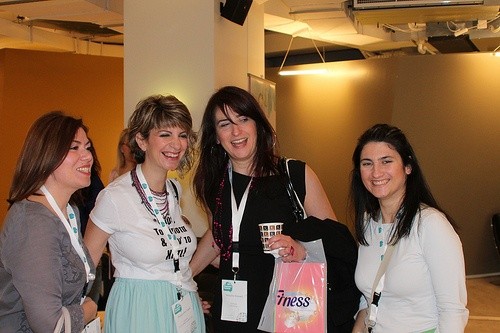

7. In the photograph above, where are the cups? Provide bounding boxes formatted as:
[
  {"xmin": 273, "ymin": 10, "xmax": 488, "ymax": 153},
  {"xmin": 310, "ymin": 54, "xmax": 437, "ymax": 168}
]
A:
[{"xmin": 258, "ymin": 222, "xmax": 284, "ymax": 254}]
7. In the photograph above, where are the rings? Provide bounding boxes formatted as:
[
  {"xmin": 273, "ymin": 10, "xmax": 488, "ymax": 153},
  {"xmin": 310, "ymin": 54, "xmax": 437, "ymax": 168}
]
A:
[{"xmin": 290, "ymin": 246, "xmax": 294, "ymax": 256}]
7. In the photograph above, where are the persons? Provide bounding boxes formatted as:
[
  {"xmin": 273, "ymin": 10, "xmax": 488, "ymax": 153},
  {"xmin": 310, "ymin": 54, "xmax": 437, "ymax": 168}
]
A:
[
  {"xmin": 347, "ymin": 123, "xmax": 469, "ymax": 333},
  {"xmin": 0, "ymin": 111, "xmax": 103, "ymax": 333},
  {"xmin": 109, "ymin": 128, "xmax": 136, "ymax": 184},
  {"xmin": 189, "ymin": 86, "xmax": 337, "ymax": 333},
  {"xmin": 84, "ymin": 95, "xmax": 209, "ymax": 333}
]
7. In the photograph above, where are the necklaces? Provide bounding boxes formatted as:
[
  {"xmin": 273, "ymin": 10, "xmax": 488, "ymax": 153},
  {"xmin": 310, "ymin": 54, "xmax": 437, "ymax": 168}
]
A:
[
  {"xmin": 212, "ymin": 168, "xmax": 256, "ymax": 260},
  {"xmin": 130, "ymin": 169, "xmax": 169, "ymax": 222}
]
